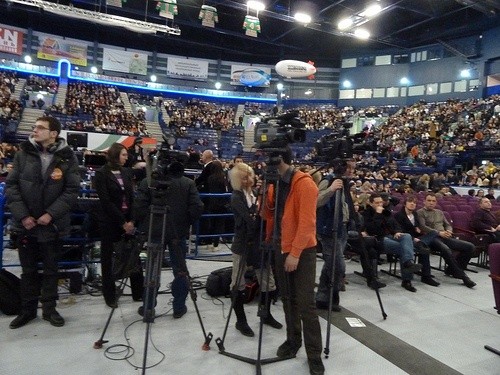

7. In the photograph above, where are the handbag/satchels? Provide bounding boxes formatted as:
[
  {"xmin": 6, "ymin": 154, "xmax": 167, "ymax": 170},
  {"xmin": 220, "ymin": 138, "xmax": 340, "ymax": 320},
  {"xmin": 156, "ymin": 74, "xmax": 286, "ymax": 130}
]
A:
[{"xmin": 108, "ymin": 228, "xmax": 144, "ymax": 281}]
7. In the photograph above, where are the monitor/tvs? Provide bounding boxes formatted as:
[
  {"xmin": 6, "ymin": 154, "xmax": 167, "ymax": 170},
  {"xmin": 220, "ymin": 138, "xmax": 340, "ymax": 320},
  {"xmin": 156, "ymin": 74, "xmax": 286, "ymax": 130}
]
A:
[{"xmin": 67, "ymin": 132, "xmax": 87, "ymax": 151}]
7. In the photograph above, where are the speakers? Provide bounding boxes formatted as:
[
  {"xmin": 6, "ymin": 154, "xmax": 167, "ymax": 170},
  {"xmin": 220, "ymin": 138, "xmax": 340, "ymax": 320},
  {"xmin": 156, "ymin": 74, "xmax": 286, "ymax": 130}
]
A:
[{"xmin": 0, "ymin": 269, "xmax": 22, "ymax": 315}]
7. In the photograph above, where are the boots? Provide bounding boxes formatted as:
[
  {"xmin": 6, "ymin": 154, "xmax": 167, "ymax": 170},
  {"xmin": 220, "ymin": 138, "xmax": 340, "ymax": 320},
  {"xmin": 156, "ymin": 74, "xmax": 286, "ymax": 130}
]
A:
[
  {"xmin": 258, "ymin": 290, "xmax": 284, "ymax": 329},
  {"xmin": 229, "ymin": 289, "xmax": 256, "ymax": 337}
]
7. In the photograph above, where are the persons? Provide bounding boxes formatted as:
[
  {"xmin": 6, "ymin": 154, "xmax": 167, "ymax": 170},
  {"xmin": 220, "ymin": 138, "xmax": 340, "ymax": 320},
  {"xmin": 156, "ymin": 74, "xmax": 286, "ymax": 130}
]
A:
[
  {"xmin": 0, "ymin": 72, "xmax": 500, "ymax": 338},
  {"xmin": 256, "ymin": 146, "xmax": 325, "ymax": 375}
]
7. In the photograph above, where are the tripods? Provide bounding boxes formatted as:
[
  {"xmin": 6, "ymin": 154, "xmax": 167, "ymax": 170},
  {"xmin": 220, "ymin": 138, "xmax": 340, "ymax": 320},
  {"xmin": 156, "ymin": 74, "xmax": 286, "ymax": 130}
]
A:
[
  {"xmin": 217, "ymin": 170, "xmax": 287, "ymax": 374},
  {"xmin": 323, "ymin": 160, "xmax": 386, "ymax": 358},
  {"xmin": 97, "ymin": 191, "xmax": 207, "ymax": 375}
]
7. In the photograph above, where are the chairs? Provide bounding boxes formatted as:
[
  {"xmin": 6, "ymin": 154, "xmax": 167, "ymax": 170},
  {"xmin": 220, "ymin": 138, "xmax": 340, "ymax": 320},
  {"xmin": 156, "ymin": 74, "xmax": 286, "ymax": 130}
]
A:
[{"xmin": 0, "ymin": 110, "xmax": 500, "ymax": 314}]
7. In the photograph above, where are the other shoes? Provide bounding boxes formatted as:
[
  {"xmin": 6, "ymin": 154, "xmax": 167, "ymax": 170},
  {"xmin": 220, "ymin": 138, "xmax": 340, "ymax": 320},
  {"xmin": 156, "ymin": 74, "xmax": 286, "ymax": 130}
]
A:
[
  {"xmin": 444, "ymin": 268, "xmax": 463, "ymax": 280},
  {"xmin": 133, "ymin": 295, "xmax": 145, "ymax": 301},
  {"xmin": 400, "ymin": 261, "xmax": 422, "ymax": 274},
  {"xmin": 366, "ymin": 280, "xmax": 386, "ymax": 290},
  {"xmin": 307, "ymin": 356, "xmax": 325, "ymax": 375},
  {"xmin": 422, "ymin": 276, "xmax": 441, "ymax": 286},
  {"xmin": 464, "ymin": 275, "xmax": 478, "ymax": 288},
  {"xmin": 104, "ymin": 297, "xmax": 118, "ymax": 308},
  {"xmin": 173, "ymin": 304, "xmax": 188, "ymax": 319},
  {"xmin": 415, "ymin": 240, "xmax": 431, "ymax": 254},
  {"xmin": 400, "ymin": 280, "xmax": 417, "ymax": 292},
  {"xmin": 317, "ymin": 301, "xmax": 342, "ymax": 311},
  {"xmin": 42, "ymin": 307, "xmax": 65, "ymax": 327},
  {"xmin": 276, "ymin": 341, "xmax": 300, "ymax": 357},
  {"xmin": 9, "ymin": 312, "xmax": 38, "ymax": 329},
  {"xmin": 137, "ymin": 306, "xmax": 156, "ymax": 319}
]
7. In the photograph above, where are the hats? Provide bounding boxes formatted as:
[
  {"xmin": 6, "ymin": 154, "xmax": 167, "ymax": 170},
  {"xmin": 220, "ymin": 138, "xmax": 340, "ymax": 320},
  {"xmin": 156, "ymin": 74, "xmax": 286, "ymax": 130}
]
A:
[{"xmin": 380, "ymin": 191, "xmax": 392, "ymax": 200}]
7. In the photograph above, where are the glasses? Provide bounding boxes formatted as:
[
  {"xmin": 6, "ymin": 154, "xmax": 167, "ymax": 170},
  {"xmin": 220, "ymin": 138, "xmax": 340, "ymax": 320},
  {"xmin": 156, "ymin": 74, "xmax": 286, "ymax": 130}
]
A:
[{"xmin": 31, "ymin": 125, "xmax": 50, "ymax": 131}]
